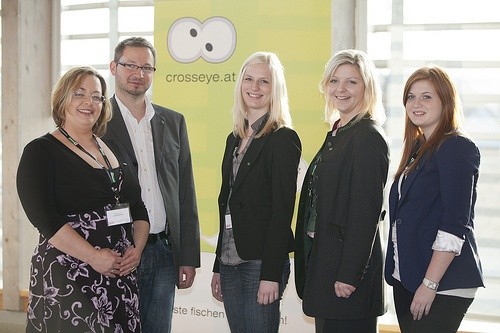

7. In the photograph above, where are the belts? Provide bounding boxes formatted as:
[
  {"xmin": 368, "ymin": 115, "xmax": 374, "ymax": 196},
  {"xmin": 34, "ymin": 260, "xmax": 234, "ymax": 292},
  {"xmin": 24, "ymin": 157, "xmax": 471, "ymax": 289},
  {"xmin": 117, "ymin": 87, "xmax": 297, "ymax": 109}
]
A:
[{"xmin": 147, "ymin": 231, "xmax": 172, "ymax": 243}]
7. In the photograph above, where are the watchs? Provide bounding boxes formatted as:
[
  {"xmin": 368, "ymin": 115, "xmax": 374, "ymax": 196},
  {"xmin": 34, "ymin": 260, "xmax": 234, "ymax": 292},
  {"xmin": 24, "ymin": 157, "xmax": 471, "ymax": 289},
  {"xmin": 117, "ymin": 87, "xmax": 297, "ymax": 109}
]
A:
[{"xmin": 422, "ymin": 277, "xmax": 439, "ymax": 290}]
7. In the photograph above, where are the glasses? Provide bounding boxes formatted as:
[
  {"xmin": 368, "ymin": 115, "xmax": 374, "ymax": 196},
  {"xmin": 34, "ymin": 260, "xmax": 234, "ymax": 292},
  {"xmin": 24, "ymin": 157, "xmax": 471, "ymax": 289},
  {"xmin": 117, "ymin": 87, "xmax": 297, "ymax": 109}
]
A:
[
  {"xmin": 116, "ymin": 62, "xmax": 156, "ymax": 72},
  {"xmin": 70, "ymin": 90, "xmax": 109, "ymax": 105}
]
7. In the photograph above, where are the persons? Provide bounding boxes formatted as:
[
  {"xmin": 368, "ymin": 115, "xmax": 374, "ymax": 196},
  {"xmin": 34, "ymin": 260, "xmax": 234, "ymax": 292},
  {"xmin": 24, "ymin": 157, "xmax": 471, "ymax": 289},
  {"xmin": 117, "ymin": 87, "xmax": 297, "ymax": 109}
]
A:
[
  {"xmin": 383, "ymin": 66, "xmax": 486, "ymax": 333},
  {"xmin": 211, "ymin": 51, "xmax": 302, "ymax": 333},
  {"xmin": 90, "ymin": 37, "xmax": 200, "ymax": 333},
  {"xmin": 294, "ymin": 49, "xmax": 389, "ymax": 333},
  {"xmin": 15, "ymin": 66, "xmax": 150, "ymax": 333}
]
